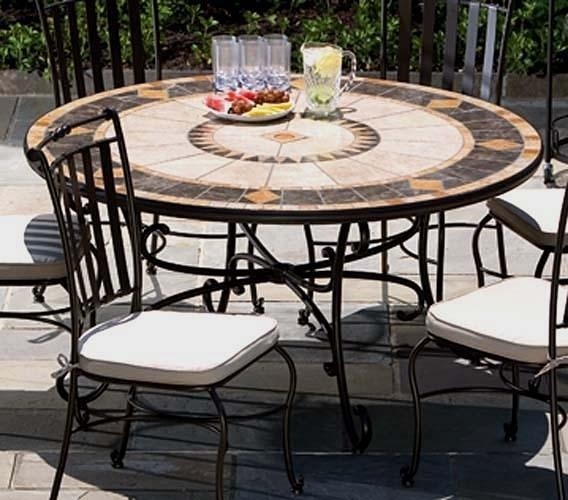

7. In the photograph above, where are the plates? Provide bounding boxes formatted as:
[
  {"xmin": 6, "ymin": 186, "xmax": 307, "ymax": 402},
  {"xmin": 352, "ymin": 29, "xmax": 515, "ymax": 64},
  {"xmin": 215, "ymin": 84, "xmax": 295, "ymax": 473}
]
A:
[{"xmin": 199, "ymin": 96, "xmax": 296, "ymax": 122}]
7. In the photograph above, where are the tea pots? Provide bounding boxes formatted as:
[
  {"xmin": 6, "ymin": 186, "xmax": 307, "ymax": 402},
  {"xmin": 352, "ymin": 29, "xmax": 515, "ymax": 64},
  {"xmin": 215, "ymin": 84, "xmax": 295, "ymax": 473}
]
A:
[{"xmin": 300, "ymin": 42, "xmax": 356, "ymax": 113}]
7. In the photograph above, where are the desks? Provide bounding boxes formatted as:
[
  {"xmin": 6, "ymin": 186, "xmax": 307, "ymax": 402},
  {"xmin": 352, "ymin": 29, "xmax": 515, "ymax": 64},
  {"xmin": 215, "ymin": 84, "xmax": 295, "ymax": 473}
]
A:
[{"xmin": 23, "ymin": 70, "xmax": 546, "ymax": 451}]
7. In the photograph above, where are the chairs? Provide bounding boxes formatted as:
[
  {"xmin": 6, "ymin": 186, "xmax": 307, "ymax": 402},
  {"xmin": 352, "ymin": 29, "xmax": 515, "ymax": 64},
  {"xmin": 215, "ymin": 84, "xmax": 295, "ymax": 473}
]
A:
[
  {"xmin": 404, "ymin": 187, "xmax": 567, "ymax": 497},
  {"xmin": 472, "ymin": 188, "xmax": 566, "ymax": 295},
  {"xmin": 1, "ymin": 214, "xmax": 101, "ymax": 342},
  {"xmin": 28, "ymin": 108, "xmax": 307, "ymax": 499}
]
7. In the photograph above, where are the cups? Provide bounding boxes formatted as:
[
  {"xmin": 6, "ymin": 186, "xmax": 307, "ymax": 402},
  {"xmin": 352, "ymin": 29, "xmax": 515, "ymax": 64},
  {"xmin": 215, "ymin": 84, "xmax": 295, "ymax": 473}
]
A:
[{"xmin": 212, "ymin": 34, "xmax": 291, "ymax": 92}]
